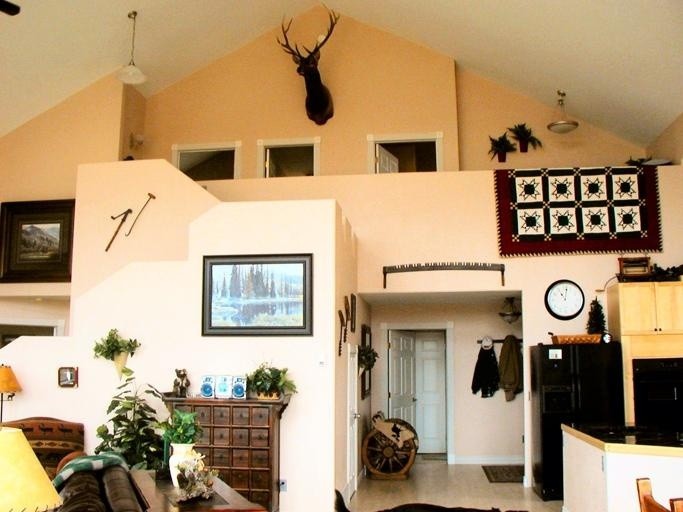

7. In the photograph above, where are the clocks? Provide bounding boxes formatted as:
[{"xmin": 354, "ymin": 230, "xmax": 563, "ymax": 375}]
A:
[{"xmin": 544, "ymin": 278, "xmax": 586, "ymax": 321}]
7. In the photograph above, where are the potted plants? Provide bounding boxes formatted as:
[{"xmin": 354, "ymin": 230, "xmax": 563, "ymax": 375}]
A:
[
  {"xmin": 159, "ymin": 406, "xmax": 205, "ymax": 489},
  {"xmin": 245, "ymin": 360, "xmax": 300, "ymax": 400},
  {"xmin": 486, "ymin": 130, "xmax": 517, "ymax": 163},
  {"xmin": 357, "ymin": 343, "xmax": 380, "ymax": 380},
  {"xmin": 506, "ymin": 122, "xmax": 543, "ymax": 152},
  {"xmin": 93, "ymin": 324, "xmax": 143, "ymax": 383}
]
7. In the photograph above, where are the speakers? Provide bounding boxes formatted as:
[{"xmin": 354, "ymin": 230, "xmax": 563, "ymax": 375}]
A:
[
  {"xmin": 232, "ymin": 375, "xmax": 247, "ymax": 399},
  {"xmin": 200, "ymin": 375, "xmax": 216, "ymax": 399}
]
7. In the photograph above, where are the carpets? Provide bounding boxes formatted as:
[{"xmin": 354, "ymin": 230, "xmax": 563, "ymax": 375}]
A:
[{"xmin": 483, "ymin": 464, "xmax": 524, "ymax": 483}]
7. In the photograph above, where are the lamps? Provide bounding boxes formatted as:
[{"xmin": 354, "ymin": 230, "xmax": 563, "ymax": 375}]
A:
[
  {"xmin": 0, "ymin": 425, "xmax": 64, "ymax": 511},
  {"xmin": 115, "ymin": 10, "xmax": 148, "ymax": 85},
  {"xmin": 497, "ymin": 295, "xmax": 520, "ymax": 325},
  {"xmin": 0, "ymin": 362, "xmax": 23, "ymax": 424},
  {"xmin": 545, "ymin": 90, "xmax": 578, "ymax": 135}
]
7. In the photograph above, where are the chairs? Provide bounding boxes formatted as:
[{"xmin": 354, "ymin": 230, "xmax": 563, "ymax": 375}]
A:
[{"xmin": 636, "ymin": 475, "xmax": 683, "ymax": 512}]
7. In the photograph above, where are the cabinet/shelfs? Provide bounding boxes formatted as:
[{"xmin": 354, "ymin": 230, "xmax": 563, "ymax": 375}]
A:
[
  {"xmin": 158, "ymin": 391, "xmax": 290, "ymax": 511},
  {"xmin": 606, "ymin": 280, "xmax": 683, "ymax": 335}
]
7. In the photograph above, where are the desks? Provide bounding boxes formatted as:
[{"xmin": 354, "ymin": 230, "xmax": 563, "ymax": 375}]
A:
[
  {"xmin": 127, "ymin": 469, "xmax": 268, "ymax": 511},
  {"xmin": 558, "ymin": 420, "xmax": 683, "ymax": 512}
]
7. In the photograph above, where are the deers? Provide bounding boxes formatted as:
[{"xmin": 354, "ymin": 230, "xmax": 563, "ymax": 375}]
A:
[{"xmin": 275, "ymin": 3, "xmax": 340, "ymax": 125}]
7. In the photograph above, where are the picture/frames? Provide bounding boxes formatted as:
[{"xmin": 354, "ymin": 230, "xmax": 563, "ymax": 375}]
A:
[
  {"xmin": 0, "ymin": 197, "xmax": 75, "ymax": 284},
  {"xmin": 360, "ymin": 321, "xmax": 373, "ymax": 402},
  {"xmin": 199, "ymin": 252, "xmax": 315, "ymax": 338}
]
